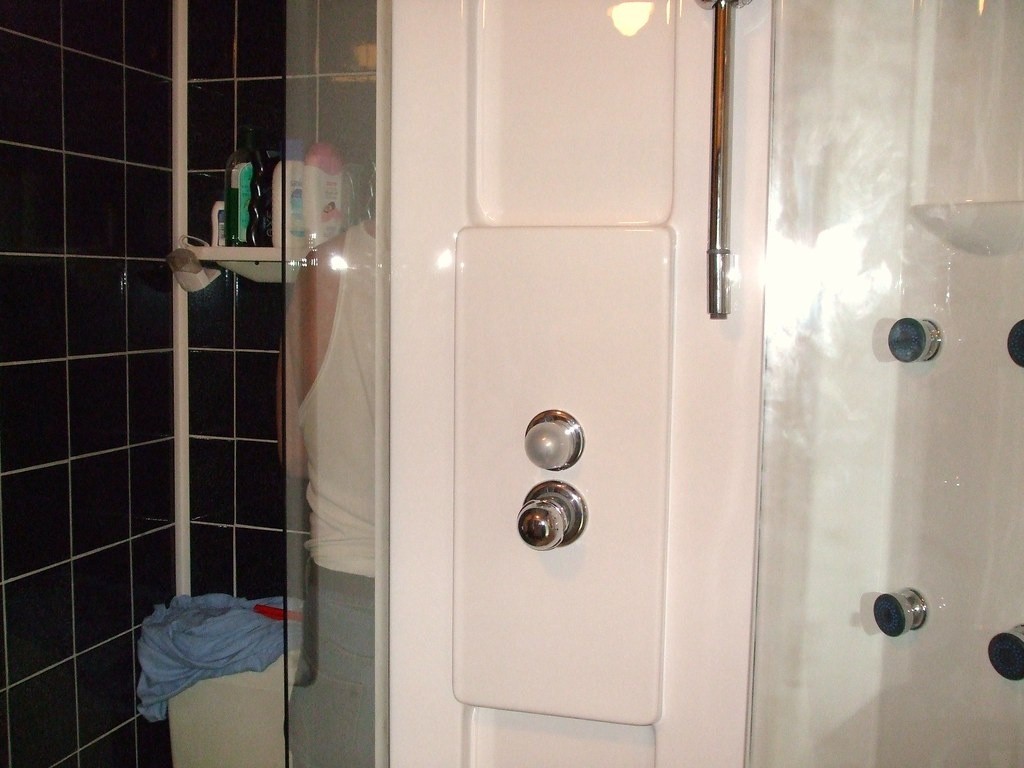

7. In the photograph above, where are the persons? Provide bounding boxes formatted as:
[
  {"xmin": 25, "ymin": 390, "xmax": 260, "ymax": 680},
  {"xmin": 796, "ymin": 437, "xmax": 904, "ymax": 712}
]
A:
[{"xmin": 277, "ymin": 149, "xmax": 377, "ymax": 768}]
